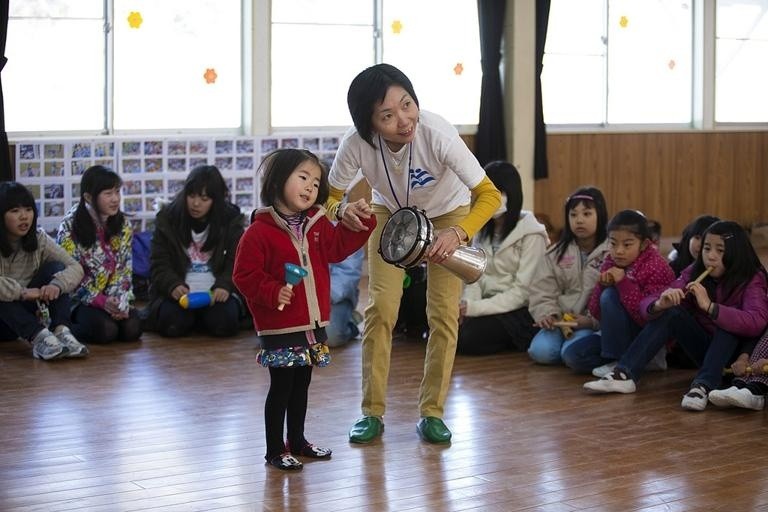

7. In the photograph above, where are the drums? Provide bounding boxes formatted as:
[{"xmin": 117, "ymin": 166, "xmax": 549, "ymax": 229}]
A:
[{"xmin": 379, "ymin": 209, "xmax": 487, "ymax": 286}]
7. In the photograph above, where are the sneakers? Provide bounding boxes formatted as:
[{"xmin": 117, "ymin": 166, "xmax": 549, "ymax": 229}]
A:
[
  {"xmin": 284, "ymin": 439, "xmax": 331, "ymax": 458},
  {"xmin": 32, "ymin": 332, "xmax": 71, "ymax": 361},
  {"xmin": 55, "ymin": 326, "xmax": 89, "ymax": 358},
  {"xmin": 265, "ymin": 447, "xmax": 303, "ymax": 471},
  {"xmin": 349, "ymin": 310, "xmax": 363, "ymax": 326},
  {"xmin": 583, "ymin": 361, "xmax": 637, "ymax": 393},
  {"xmin": 681, "ymin": 386, "xmax": 765, "ymax": 411}
]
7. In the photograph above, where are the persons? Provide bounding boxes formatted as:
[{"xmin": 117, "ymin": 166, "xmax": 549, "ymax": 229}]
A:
[
  {"xmin": 457, "ymin": 161, "xmax": 551, "ymax": 355},
  {"xmin": 321, "ymin": 63, "xmax": 501, "ymax": 447},
  {"xmin": 56, "ymin": 164, "xmax": 143, "ymax": 346},
  {"xmin": 230, "ymin": 149, "xmax": 377, "ymax": 470},
  {"xmin": 135, "ymin": 165, "xmax": 251, "ymax": 338},
  {"xmin": 527, "ymin": 188, "xmax": 768, "ymax": 410},
  {"xmin": 1, "ymin": 181, "xmax": 91, "ymax": 362},
  {"xmin": 320, "ymin": 158, "xmax": 363, "ymax": 349}
]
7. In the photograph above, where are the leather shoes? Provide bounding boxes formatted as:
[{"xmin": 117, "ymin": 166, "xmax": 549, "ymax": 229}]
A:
[
  {"xmin": 349, "ymin": 416, "xmax": 384, "ymax": 444},
  {"xmin": 416, "ymin": 417, "xmax": 451, "ymax": 444}
]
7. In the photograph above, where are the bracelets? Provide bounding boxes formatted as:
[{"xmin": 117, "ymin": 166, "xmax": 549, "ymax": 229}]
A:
[{"xmin": 333, "ymin": 202, "xmax": 345, "ymax": 221}]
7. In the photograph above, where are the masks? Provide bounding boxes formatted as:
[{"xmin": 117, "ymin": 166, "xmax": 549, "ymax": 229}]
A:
[{"xmin": 491, "ymin": 195, "xmax": 507, "ymax": 219}]
